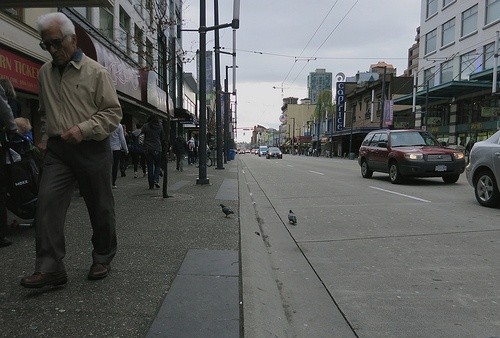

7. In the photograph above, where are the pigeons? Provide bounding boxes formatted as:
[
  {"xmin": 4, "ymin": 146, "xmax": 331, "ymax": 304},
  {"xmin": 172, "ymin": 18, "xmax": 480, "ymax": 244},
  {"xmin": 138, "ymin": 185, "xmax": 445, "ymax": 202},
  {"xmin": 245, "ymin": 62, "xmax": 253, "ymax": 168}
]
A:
[
  {"xmin": 288, "ymin": 209, "xmax": 298, "ymax": 226},
  {"xmin": 219, "ymin": 203, "xmax": 235, "ymax": 218}
]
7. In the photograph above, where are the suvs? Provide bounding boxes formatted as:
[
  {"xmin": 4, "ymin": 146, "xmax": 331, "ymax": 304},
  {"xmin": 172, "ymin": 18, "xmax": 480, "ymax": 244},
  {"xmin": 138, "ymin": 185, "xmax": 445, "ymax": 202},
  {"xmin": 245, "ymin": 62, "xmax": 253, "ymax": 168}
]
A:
[
  {"xmin": 259, "ymin": 146, "xmax": 268, "ymax": 157},
  {"xmin": 357, "ymin": 128, "xmax": 467, "ymax": 184}
]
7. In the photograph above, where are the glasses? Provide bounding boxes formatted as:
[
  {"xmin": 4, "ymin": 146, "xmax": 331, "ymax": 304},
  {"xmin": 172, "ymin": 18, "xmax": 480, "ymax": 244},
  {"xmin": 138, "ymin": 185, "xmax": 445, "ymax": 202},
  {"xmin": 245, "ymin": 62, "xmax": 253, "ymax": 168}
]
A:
[{"xmin": 39, "ymin": 35, "xmax": 67, "ymax": 51}]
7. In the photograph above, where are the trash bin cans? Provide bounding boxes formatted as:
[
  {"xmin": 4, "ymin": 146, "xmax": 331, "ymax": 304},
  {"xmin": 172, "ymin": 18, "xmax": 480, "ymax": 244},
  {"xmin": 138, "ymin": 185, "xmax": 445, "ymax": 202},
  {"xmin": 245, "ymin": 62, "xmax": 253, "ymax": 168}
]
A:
[{"xmin": 230, "ymin": 149, "xmax": 236, "ymax": 160}]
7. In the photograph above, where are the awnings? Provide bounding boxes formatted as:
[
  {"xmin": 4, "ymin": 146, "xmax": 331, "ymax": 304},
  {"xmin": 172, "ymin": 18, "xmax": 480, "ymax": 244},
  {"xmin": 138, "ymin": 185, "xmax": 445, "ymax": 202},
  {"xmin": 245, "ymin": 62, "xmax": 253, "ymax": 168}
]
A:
[{"xmin": 393, "ymin": 65, "xmax": 500, "ymax": 105}]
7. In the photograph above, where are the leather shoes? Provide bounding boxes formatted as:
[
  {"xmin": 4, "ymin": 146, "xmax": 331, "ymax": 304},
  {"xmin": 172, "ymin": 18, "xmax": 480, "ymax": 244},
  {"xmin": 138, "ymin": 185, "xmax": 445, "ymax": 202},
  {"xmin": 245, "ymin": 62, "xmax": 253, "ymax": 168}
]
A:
[
  {"xmin": 20, "ymin": 270, "xmax": 69, "ymax": 289},
  {"xmin": 88, "ymin": 262, "xmax": 112, "ymax": 279}
]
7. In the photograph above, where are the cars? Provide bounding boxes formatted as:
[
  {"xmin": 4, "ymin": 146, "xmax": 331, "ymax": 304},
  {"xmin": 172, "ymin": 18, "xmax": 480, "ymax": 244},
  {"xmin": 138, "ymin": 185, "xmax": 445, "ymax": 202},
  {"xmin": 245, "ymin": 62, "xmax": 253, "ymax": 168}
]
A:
[
  {"xmin": 466, "ymin": 129, "xmax": 500, "ymax": 207},
  {"xmin": 235, "ymin": 148, "xmax": 259, "ymax": 155},
  {"xmin": 266, "ymin": 147, "xmax": 282, "ymax": 159}
]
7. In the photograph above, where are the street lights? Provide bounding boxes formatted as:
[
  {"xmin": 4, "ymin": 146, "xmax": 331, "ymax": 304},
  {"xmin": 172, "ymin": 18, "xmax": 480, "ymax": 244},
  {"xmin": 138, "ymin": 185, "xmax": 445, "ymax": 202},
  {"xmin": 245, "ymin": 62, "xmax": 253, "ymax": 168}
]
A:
[{"xmin": 225, "ymin": 65, "xmax": 238, "ymax": 161}]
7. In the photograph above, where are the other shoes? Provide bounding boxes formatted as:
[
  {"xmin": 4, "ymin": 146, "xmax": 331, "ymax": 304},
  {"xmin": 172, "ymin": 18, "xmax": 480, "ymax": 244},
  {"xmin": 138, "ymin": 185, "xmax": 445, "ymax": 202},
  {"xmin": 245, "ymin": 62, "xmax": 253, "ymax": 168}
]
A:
[
  {"xmin": 149, "ymin": 183, "xmax": 155, "ymax": 190},
  {"xmin": 133, "ymin": 171, "xmax": 138, "ymax": 178},
  {"xmin": 112, "ymin": 185, "xmax": 118, "ymax": 189},
  {"xmin": 153, "ymin": 180, "xmax": 161, "ymax": 189}
]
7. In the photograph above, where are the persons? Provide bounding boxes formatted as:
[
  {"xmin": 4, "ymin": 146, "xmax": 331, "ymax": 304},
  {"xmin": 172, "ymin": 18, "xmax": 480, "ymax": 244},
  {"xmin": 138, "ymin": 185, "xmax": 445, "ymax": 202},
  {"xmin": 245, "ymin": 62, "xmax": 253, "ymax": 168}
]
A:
[
  {"xmin": 19, "ymin": 12, "xmax": 123, "ymax": 288},
  {"xmin": 160, "ymin": 132, "xmax": 199, "ymax": 177},
  {"xmin": 292, "ymin": 146, "xmax": 319, "ymax": 157},
  {"xmin": 0, "ymin": 79, "xmax": 33, "ymax": 248},
  {"xmin": 459, "ymin": 139, "xmax": 476, "ymax": 152},
  {"xmin": 142, "ymin": 115, "xmax": 168, "ymax": 188},
  {"xmin": 111, "ymin": 121, "xmax": 148, "ymax": 188}
]
